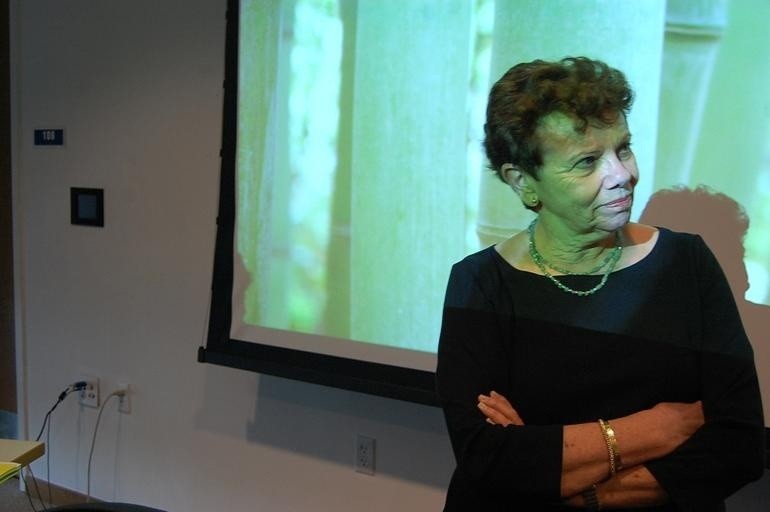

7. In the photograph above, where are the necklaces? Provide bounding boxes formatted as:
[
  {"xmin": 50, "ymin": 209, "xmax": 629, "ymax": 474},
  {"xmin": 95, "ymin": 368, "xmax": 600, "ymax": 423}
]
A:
[{"xmin": 526, "ymin": 219, "xmax": 625, "ymax": 296}]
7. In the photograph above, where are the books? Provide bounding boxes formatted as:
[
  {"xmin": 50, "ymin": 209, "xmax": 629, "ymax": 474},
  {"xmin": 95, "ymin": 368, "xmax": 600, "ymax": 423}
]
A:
[{"xmin": 0, "ymin": 462, "xmax": 21, "ymax": 485}]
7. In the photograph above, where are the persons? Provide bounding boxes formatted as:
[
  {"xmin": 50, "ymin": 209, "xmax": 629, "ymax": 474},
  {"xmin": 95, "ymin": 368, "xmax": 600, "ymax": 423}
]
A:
[{"xmin": 437, "ymin": 53, "xmax": 765, "ymax": 512}]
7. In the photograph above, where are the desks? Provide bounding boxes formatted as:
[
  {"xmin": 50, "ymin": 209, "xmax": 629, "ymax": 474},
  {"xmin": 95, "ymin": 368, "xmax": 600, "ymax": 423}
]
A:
[{"xmin": 0, "ymin": 439, "xmax": 45, "ymax": 468}]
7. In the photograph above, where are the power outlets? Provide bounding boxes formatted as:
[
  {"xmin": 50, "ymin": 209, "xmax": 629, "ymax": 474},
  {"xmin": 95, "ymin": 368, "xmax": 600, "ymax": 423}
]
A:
[
  {"xmin": 77, "ymin": 375, "xmax": 100, "ymax": 408},
  {"xmin": 355, "ymin": 436, "xmax": 376, "ymax": 476},
  {"xmin": 117, "ymin": 384, "xmax": 131, "ymax": 413}
]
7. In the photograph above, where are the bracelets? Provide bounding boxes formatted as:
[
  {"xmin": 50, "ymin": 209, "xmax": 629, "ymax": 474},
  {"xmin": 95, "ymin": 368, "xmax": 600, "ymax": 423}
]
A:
[{"xmin": 598, "ymin": 418, "xmax": 623, "ymax": 478}]
